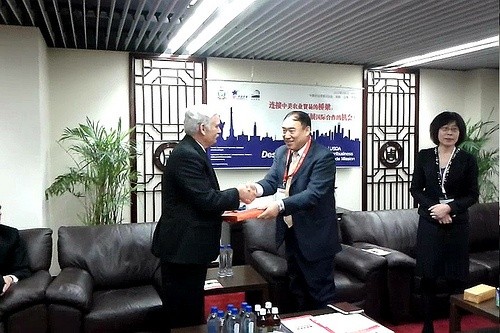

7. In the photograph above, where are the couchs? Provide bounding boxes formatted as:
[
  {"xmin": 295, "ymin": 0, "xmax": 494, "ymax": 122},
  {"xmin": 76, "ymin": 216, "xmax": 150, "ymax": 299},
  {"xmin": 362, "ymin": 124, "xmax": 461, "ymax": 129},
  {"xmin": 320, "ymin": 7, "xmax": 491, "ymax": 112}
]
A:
[
  {"xmin": 238, "ymin": 214, "xmax": 390, "ymax": 321},
  {"xmin": 0, "ymin": 227, "xmax": 54, "ymax": 333},
  {"xmin": 47, "ymin": 222, "xmax": 165, "ymax": 333},
  {"xmin": 340, "ymin": 202, "xmax": 500, "ymax": 319}
]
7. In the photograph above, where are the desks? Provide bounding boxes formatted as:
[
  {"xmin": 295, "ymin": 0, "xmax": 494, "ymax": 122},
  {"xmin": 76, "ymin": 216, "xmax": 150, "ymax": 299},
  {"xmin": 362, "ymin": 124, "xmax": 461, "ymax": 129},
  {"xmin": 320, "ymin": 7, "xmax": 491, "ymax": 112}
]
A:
[
  {"xmin": 171, "ymin": 308, "xmax": 394, "ymax": 333},
  {"xmin": 449, "ymin": 294, "xmax": 500, "ymax": 333},
  {"xmin": 204, "ymin": 264, "xmax": 269, "ymax": 309}
]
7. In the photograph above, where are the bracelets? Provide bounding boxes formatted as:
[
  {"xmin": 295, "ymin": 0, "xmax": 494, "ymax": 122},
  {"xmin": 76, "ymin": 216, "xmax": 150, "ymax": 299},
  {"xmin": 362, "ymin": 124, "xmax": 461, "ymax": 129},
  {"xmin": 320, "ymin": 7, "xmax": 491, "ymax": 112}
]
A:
[
  {"xmin": 277, "ymin": 202, "xmax": 284, "ymax": 214},
  {"xmin": 8, "ymin": 276, "xmax": 13, "ymax": 283}
]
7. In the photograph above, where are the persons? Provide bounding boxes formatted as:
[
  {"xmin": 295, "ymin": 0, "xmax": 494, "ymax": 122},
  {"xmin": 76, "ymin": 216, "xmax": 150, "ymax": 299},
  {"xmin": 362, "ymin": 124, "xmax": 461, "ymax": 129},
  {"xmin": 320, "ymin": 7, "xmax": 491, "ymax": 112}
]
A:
[
  {"xmin": 245, "ymin": 111, "xmax": 343, "ymax": 312},
  {"xmin": 150, "ymin": 103, "xmax": 257, "ymax": 333},
  {"xmin": 411, "ymin": 112, "xmax": 479, "ymax": 333},
  {"xmin": 0, "ymin": 205, "xmax": 33, "ymax": 296}
]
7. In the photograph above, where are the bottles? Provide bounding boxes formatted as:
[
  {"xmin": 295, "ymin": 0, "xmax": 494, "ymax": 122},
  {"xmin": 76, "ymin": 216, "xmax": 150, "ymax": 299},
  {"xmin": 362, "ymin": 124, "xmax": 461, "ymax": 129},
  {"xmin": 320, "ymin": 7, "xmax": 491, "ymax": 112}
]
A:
[
  {"xmin": 241, "ymin": 306, "xmax": 255, "ymax": 333},
  {"xmin": 255, "ymin": 308, "xmax": 267, "ymax": 333},
  {"xmin": 227, "ymin": 309, "xmax": 242, "ymax": 333},
  {"xmin": 238, "ymin": 302, "xmax": 248, "ymax": 318},
  {"xmin": 226, "ymin": 244, "xmax": 233, "ymax": 276},
  {"xmin": 265, "ymin": 302, "xmax": 273, "ymax": 332},
  {"xmin": 271, "ymin": 307, "xmax": 281, "ymax": 333},
  {"xmin": 217, "ymin": 311, "xmax": 226, "ymax": 331},
  {"xmin": 207, "ymin": 307, "xmax": 221, "ymax": 333},
  {"xmin": 218, "ymin": 245, "xmax": 227, "ymax": 278},
  {"xmin": 255, "ymin": 304, "xmax": 261, "ymax": 319},
  {"xmin": 225, "ymin": 304, "xmax": 234, "ymax": 321}
]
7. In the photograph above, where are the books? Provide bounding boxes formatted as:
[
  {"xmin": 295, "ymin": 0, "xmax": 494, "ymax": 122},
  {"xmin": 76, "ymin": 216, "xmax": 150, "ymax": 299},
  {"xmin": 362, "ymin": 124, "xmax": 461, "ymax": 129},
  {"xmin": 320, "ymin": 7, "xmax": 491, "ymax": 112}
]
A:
[
  {"xmin": 280, "ymin": 314, "xmax": 336, "ymax": 332},
  {"xmin": 326, "ymin": 301, "xmax": 364, "ymax": 315},
  {"xmin": 313, "ymin": 311, "xmax": 396, "ymax": 332}
]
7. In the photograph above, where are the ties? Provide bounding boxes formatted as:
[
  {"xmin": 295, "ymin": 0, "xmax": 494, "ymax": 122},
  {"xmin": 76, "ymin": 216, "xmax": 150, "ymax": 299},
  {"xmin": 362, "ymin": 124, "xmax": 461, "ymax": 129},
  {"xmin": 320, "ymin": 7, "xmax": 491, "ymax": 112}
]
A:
[{"xmin": 283, "ymin": 151, "xmax": 298, "ymax": 228}]
